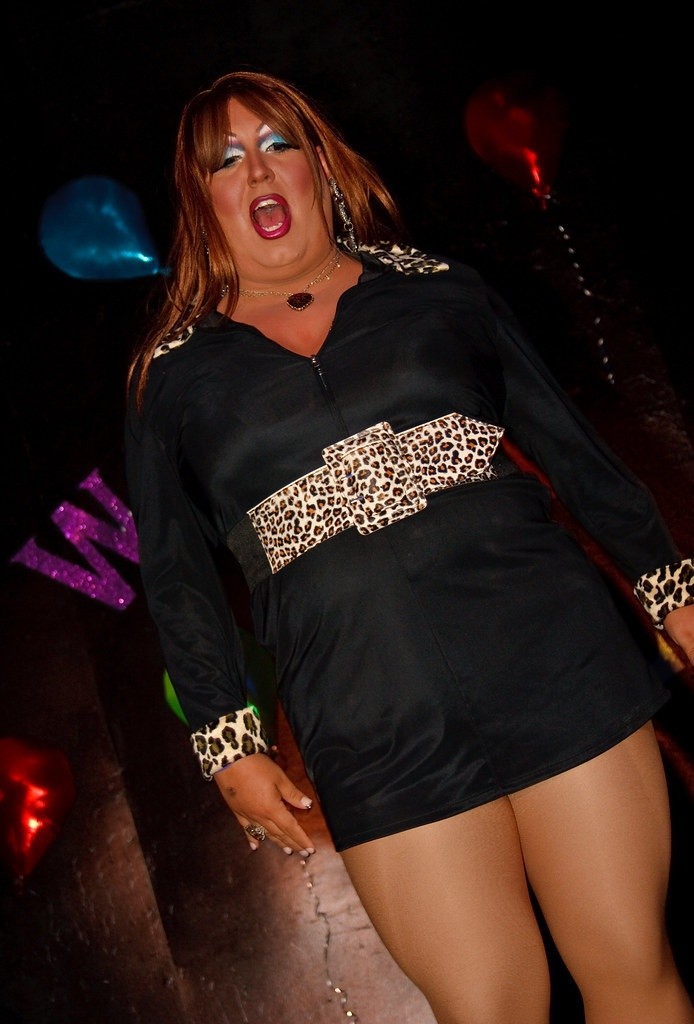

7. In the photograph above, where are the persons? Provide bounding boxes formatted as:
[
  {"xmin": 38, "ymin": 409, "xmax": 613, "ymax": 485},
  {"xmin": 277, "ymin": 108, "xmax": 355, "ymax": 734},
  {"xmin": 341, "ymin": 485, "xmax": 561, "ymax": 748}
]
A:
[{"xmin": 126, "ymin": 75, "xmax": 694, "ymax": 1024}]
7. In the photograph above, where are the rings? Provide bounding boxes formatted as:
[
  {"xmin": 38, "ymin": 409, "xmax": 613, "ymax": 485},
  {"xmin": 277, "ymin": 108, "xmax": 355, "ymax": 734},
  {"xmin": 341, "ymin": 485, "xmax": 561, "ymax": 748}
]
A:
[{"xmin": 245, "ymin": 824, "xmax": 265, "ymax": 841}]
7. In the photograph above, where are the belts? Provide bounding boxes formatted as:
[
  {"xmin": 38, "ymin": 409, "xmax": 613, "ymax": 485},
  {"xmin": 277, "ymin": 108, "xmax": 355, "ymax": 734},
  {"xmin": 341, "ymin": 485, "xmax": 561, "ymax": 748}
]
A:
[{"xmin": 223, "ymin": 412, "xmax": 512, "ymax": 595}]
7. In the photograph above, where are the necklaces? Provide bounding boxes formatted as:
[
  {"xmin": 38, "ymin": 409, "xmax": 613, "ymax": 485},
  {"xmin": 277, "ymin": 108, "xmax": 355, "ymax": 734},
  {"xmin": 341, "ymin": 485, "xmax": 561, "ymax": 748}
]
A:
[{"xmin": 223, "ymin": 248, "xmax": 341, "ymax": 310}]
